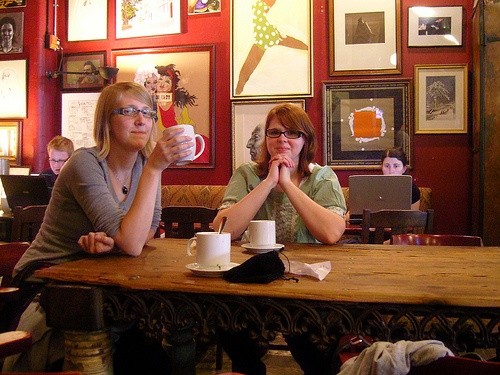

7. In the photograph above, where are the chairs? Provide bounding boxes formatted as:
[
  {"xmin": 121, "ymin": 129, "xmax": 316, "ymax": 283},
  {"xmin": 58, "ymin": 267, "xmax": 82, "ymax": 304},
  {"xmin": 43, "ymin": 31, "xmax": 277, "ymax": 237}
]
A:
[{"xmin": 0, "ymin": 207, "xmax": 484, "ymax": 375}]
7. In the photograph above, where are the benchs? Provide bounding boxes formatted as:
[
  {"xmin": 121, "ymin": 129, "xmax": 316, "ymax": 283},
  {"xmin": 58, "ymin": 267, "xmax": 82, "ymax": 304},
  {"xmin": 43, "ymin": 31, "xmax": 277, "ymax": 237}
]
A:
[{"xmin": 156, "ymin": 184, "xmax": 434, "ymax": 235}]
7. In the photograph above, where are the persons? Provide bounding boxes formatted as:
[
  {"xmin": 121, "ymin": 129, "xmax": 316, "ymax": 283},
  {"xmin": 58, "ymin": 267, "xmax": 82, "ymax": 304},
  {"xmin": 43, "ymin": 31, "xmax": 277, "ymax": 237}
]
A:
[
  {"xmin": 38, "ymin": 135, "xmax": 74, "ymax": 187},
  {"xmin": 212, "ymin": 102, "xmax": 348, "ymax": 375},
  {"xmin": 380, "ymin": 145, "xmax": 420, "ymax": 211},
  {"xmin": 0, "ymin": 177, "xmax": 13, "ymax": 217},
  {"xmin": 10, "ymin": 81, "xmax": 197, "ymax": 375}
]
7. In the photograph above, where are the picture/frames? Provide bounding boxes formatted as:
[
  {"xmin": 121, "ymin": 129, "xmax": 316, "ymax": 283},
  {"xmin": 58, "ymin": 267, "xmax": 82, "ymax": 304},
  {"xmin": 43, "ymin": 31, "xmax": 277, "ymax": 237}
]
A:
[
  {"xmin": 229, "ymin": 0, "xmax": 315, "ymax": 99},
  {"xmin": 407, "ymin": 6, "xmax": 463, "ymax": 48},
  {"xmin": 0, "ymin": 59, "xmax": 28, "ymax": 119},
  {"xmin": 320, "ymin": 77, "xmax": 415, "ymax": 172},
  {"xmin": 109, "ymin": 44, "xmax": 216, "ymax": 169},
  {"xmin": 327, "ymin": 0, "xmax": 401, "ymax": 77},
  {"xmin": 0, "ymin": 120, "xmax": 22, "ymax": 166},
  {"xmin": 230, "ymin": 99, "xmax": 306, "ymax": 176},
  {"xmin": 412, "ymin": 62, "xmax": 468, "ymax": 134},
  {"xmin": 59, "ymin": 50, "xmax": 105, "ymax": 89}
]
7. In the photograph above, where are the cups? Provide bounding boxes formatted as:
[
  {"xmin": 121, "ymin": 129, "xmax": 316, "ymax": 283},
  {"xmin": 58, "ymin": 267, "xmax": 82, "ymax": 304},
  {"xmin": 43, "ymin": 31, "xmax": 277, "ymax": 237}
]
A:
[
  {"xmin": 249, "ymin": 219, "xmax": 278, "ymax": 249},
  {"xmin": 187, "ymin": 231, "xmax": 231, "ymax": 270},
  {"xmin": 163, "ymin": 124, "xmax": 206, "ymax": 166},
  {"xmin": 0, "ymin": 158, "xmax": 9, "ymax": 176}
]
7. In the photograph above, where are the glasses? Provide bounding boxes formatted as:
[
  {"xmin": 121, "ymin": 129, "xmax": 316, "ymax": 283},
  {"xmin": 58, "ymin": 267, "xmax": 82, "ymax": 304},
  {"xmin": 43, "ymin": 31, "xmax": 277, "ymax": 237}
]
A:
[
  {"xmin": 112, "ymin": 107, "xmax": 156, "ymax": 118},
  {"xmin": 49, "ymin": 156, "xmax": 70, "ymax": 165},
  {"xmin": 266, "ymin": 129, "xmax": 302, "ymax": 139}
]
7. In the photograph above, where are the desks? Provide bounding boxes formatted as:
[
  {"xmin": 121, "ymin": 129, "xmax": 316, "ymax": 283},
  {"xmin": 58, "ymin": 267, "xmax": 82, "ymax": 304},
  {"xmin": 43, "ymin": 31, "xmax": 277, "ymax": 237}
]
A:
[{"xmin": 32, "ymin": 239, "xmax": 500, "ymax": 375}]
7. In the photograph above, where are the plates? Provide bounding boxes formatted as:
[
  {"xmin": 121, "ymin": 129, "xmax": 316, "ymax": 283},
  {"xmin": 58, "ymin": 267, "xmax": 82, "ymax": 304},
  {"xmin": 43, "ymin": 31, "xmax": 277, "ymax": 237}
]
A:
[
  {"xmin": 185, "ymin": 263, "xmax": 240, "ymax": 277},
  {"xmin": 242, "ymin": 242, "xmax": 286, "ymax": 254}
]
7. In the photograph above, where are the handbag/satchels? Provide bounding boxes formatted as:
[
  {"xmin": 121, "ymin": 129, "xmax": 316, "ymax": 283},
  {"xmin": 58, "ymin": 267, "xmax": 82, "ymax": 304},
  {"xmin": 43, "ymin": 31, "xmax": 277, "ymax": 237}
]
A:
[{"xmin": 224, "ymin": 250, "xmax": 298, "ymax": 285}]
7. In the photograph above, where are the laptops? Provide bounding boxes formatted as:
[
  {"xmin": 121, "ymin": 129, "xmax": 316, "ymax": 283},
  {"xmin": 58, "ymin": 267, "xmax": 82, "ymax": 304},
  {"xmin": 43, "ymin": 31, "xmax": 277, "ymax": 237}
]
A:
[{"xmin": 347, "ymin": 174, "xmax": 412, "ymax": 225}]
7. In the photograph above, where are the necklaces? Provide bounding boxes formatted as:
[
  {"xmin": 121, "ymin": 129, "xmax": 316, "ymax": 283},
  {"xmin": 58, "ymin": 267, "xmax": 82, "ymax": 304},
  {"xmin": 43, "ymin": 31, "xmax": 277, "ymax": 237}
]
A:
[{"xmin": 114, "ymin": 173, "xmax": 130, "ymax": 194}]
